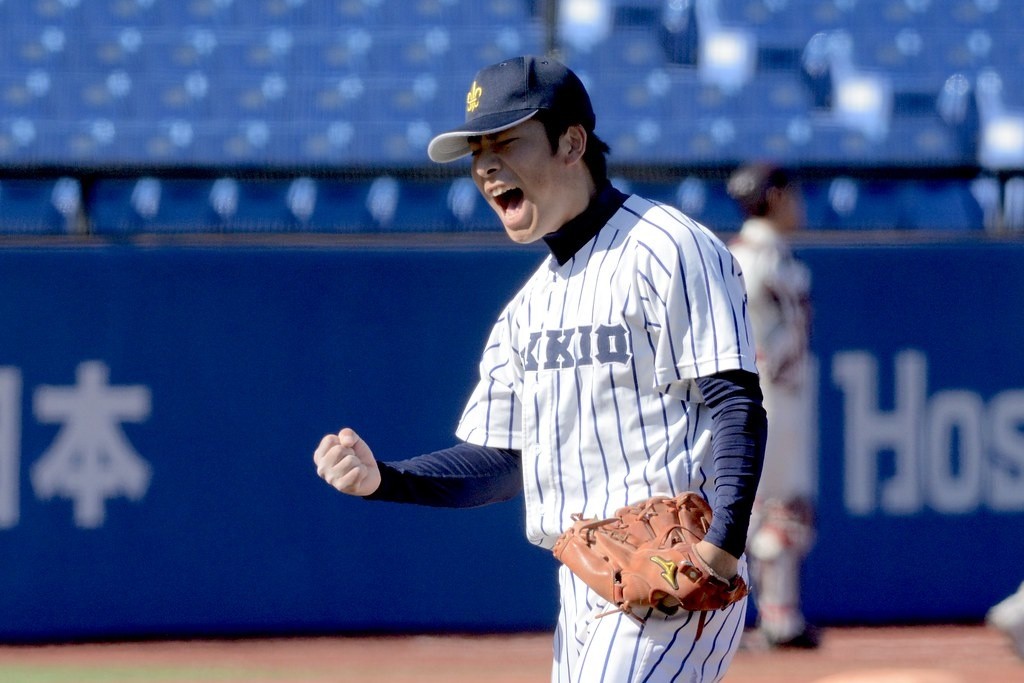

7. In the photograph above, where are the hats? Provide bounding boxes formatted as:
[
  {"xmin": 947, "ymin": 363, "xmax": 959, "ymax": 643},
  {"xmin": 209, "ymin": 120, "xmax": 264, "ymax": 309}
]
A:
[{"xmin": 427, "ymin": 55, "xmax": 596, "ymax": 164}]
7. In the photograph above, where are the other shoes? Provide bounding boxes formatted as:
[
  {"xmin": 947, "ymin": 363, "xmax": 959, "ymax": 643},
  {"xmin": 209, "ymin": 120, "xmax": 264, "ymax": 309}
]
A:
[{"xmin": 766, "ymin": 628, "xmax": 818, "ymax": 650}]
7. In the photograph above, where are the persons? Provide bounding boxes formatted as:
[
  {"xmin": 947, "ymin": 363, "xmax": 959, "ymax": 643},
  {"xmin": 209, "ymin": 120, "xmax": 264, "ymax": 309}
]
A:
[
  {"xmin": 313, "ymin": 55, "xmax": 770, "ymax": 683},
  {"xmin": 724, "ymin": 163, "xmax": 822, "ymax": 651}
]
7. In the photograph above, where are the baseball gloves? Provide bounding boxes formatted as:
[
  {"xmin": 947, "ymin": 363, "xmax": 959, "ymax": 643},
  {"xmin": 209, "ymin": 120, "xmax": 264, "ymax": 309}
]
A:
[{"xmin": 551, "ymin": 490, "xmax": 749, "ymax": 642}]
